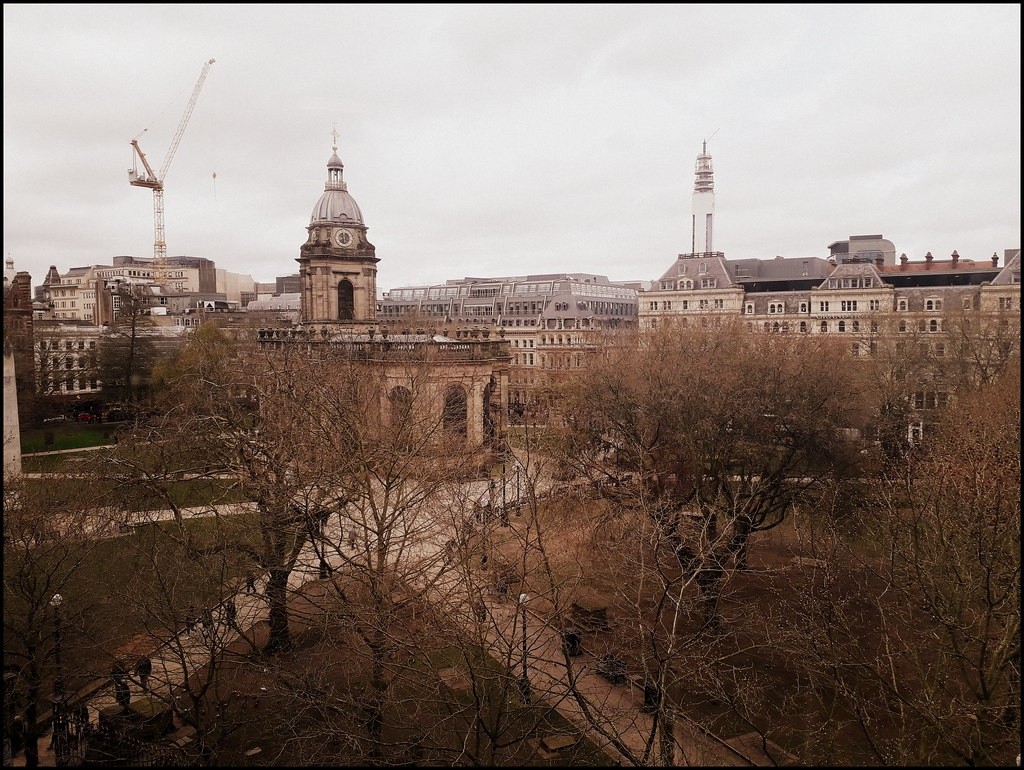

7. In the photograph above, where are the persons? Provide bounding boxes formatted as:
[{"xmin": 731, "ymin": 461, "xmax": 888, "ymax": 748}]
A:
[
  {"xmin": 185, "ymin": 600, "xmax": 239, "ymax": 641},
  {"xmin": 107, "ymin": 654, "xmax": 152, "ymax": 704},
  {"xmin": 11, "ymin": 705, "xmax": 90, "ymax": 758},
  {"xmin": 244, "ymin": 567, "xmax": 257, "ymax": 597},
  {"xmin": 348, "ymin": 528, "xmax": 358, "ymax": 550}
]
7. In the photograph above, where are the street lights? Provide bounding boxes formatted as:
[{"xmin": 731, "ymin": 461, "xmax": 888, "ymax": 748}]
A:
[
  {"xmin": 514, "ymin": 460, "xmax": 521, "ymax": 517},
  {"xmin": 49, "ymin": 594, "xmax": 63, "ymax": 696},
  {"xmin": 517, "ymin": 594, "xmax": 531, "ymax": 706}
]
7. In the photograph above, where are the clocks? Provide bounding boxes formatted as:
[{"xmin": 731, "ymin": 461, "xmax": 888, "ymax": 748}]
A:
[{"xmin": 334, "ymin": 229, "xmax": 354, "ymax": 248}]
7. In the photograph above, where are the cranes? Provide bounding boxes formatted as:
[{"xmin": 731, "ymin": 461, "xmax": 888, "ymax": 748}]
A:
[{"xmin": 128, "ymin": 57, "xmax": 216, "ymax": 284}]
